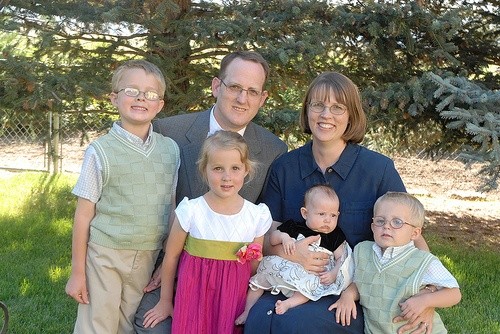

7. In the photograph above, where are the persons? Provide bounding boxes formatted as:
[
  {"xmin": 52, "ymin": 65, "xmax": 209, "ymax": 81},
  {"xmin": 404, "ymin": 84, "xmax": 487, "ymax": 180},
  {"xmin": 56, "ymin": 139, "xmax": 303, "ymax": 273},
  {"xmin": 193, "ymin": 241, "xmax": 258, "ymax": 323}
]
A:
[
  {"xmin": 63, "ymin": 59, "xmax": 181, "ymax": 334},
  {"xmin": 139, "ymin": 49, "xmax": 290, "ymax": 334},
  {"xmin": 328, "ymin": 192, "xmax": 461, "ymax": 334},
  {"xmin": 143, "ymin": 130, "xmax": 275, "ymax": 334},
  {"xmin": 244, "ymin": 74, "xmax": 431, "ymax": 334},
  {"xmin": 233, "ymin": 185, "xmax": 350, "ymax": 326}
]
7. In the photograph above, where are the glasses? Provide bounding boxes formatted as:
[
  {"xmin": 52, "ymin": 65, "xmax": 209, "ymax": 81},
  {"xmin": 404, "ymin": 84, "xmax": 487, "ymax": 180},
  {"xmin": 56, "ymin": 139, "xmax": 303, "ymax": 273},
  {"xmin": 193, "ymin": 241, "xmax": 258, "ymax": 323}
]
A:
[
  {"xmin": 307, "ymin": 101, "xmax": 348, "ymax": 116},
  {"xmin": 372, "ymin": 216, "xmax": 417, "ymax": 230},
  {"xmin": 220, "ymin": 78, "xmax": 263, "ymax": 99},
  {"xmin": 116, "ymin": 88, "xmax": 162, "ymax": 101}
]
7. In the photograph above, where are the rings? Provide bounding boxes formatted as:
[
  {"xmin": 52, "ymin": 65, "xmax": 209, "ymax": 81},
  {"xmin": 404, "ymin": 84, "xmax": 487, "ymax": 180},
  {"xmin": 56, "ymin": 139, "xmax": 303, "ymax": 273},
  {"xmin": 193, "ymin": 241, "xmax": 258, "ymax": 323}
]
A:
[{"xmin": 423, "ymin": 323, "xmax": 428, "ymax": 328}]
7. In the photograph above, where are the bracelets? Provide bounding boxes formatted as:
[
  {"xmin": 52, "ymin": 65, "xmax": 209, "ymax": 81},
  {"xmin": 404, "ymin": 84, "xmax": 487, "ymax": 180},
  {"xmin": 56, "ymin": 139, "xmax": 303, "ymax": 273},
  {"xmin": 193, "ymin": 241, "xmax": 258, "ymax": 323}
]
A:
[{"xmin": 425, "ymin": 286, "xmax": 435, "ymax": 293}]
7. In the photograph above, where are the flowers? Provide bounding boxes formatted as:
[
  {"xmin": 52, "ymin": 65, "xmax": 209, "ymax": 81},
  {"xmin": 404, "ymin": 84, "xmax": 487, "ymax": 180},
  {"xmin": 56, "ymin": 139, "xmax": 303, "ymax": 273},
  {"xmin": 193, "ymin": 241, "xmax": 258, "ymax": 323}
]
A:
[{"xmin": 236, "ymin": 242, "xmax": 263, "ymax": 264}]
